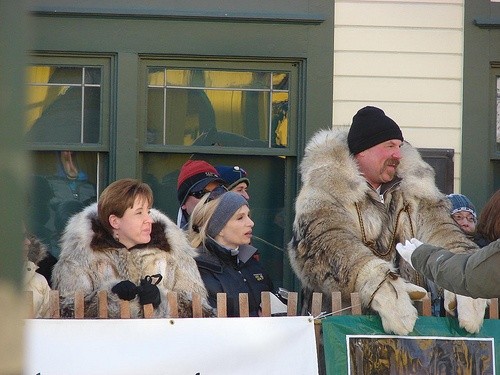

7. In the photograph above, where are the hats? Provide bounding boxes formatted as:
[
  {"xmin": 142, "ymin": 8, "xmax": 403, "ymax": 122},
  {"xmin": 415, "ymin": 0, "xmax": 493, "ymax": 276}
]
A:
[
  {"xmin": 212, "ymin": 164, "xmax": 250, "ymax": 190},
  {"xmin": 442, "ymin": 192, "xmax": 476, "ymax": 218},
  {"xmin": 347, "ymin": 105, "xmax": 403, "ymax": 154},
  {"xmin": 177, "ymin": 160, "xmax": 227, "ymax": 206}
]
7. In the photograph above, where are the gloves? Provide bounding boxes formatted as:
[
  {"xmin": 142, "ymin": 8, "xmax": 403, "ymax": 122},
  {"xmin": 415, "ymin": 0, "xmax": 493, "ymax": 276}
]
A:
[
  {"xmin": 443, "ymin": 287, "xmax": 492, "ymax": 334},
  {"xmin": 396, "ymin": 238, "xmax": 424, "ymax": 272},
  {"xmin": 137, "ymin": 284, "xmax": 162, "ymax": 308},
  {"xmin": 370, "ymin": 274, "xmax": 429, "ymax": 336},
  {"xmin": 111, "ymin": 280, "xmax": 137, "ymax": 301}
]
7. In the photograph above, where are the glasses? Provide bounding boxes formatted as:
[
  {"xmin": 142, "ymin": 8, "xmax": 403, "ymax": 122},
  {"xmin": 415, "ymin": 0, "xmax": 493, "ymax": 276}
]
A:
[
  {"xmin": 204, "ymin": 184, "xmax": 229, "ymax": 204},
  {"xmin": 451, "ymin": 213, "xmax": 474, "ymax": 222},
  {"xmin": 188, "ymin": 189, "xmax": 212, "ymax": 199}
]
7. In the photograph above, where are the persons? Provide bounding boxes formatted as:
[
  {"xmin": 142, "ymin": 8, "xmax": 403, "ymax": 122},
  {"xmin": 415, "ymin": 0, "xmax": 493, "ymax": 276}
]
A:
[
  {"xmin": 22, "ymin": 162, "xmax": 291, "ymax": 319},
  {"xmin": 289, "ymin": 104, "xmax": 489, "ymax": 334},
  {"xmin": 396, "ymin": 192, "xmax": 500, "ymax": 322},
  {"xmin": 33, "ymin": 151, "xmax": 107, "ymax": 257}
]
